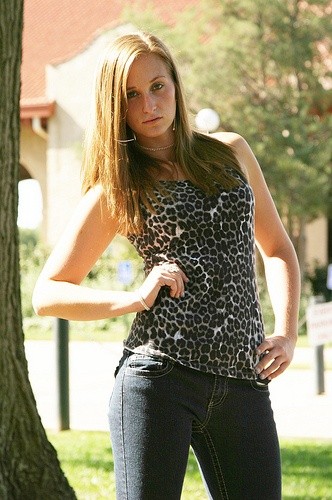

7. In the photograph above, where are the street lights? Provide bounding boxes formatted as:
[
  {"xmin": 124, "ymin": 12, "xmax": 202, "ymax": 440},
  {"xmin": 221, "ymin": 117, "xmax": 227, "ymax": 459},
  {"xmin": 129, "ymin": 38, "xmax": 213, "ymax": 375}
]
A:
[{"xmin": 196, "ymin": 108, "xmax": 220, "ymax": 134}]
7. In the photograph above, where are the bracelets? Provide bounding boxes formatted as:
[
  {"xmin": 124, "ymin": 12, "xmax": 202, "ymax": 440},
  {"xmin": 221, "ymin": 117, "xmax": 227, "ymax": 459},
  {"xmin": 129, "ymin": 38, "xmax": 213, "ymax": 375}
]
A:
[{"xmin": 135, "ymin": 290, "xmax": 150, "ymax": 311}]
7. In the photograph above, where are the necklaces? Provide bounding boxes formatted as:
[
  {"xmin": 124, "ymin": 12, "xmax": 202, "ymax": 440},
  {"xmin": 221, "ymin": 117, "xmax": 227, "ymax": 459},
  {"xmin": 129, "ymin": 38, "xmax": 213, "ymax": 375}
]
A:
[{"xmin": 133, "ymin": 142, "xmax": 176, "ymax": 151}]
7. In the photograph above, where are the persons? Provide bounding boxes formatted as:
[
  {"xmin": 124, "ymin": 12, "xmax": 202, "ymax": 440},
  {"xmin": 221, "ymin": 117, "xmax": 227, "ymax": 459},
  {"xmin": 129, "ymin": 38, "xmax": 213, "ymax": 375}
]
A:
[{"xmin": 32, "ymin": 32, "xmax": 301, "ymax": 500}]
